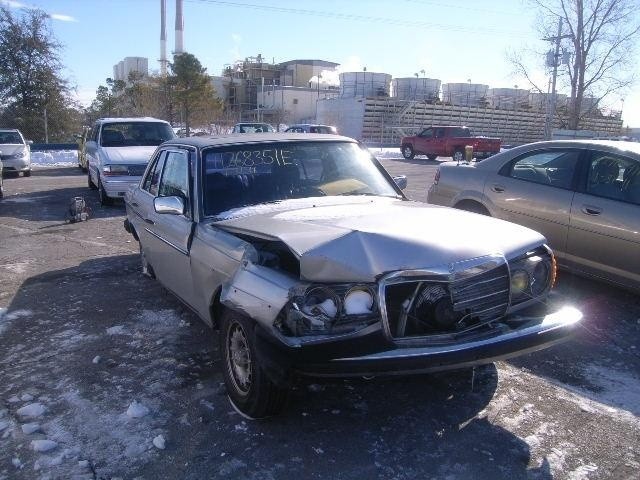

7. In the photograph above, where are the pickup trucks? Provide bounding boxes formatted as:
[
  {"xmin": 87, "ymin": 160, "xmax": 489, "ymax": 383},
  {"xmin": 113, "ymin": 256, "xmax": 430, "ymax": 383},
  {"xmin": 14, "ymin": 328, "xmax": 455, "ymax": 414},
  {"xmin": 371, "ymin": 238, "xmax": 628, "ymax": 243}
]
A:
[{"xmin": 401, "ymin": 124, "xmax": 503, "ymax": 164}]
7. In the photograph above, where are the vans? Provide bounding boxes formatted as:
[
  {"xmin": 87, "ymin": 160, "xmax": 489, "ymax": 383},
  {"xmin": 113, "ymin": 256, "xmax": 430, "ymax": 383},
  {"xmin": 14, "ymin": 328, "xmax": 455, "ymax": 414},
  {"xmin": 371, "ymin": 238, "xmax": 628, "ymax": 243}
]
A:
[{"xmin": 590, "ymin": 136, "xmax": 639, "ymax": 144}]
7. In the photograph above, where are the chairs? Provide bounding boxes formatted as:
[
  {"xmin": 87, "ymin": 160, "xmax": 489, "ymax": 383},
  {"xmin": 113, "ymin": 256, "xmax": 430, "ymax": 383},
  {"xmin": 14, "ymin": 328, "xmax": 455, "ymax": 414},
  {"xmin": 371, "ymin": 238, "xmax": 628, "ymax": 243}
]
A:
[
  {"xmin": 590, "ymin": 159, "xmax": 629, "ymax": 203},
  {"xmin": 101, "ymin": 130, "xmax": 127, "ymax": 146},
  {"xmin": 7, "ymin": 134, "xmax": 18, "ymax": 144},
  {"xmin": 270, "ymin": 158, "xmax": 311, "ymax": 200},
  {"xmin": 206, "ymin": 172, "xmax": 245, "ymax": 214},
  {"xmin": 622, "ymin": 162, "xmax": 640, "ymax": 206}
]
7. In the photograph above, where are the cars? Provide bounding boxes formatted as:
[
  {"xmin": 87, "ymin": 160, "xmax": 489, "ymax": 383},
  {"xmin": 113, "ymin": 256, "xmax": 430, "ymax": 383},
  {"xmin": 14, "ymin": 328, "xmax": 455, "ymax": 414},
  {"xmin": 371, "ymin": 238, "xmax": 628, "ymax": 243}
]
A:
[
  {"xmin": 121, "ymin": 130, "xmax": 587, "ymax": 435},
  {"xmin": 423, "ymin": 136, "xmax": 640, "ymax": 301}
]
